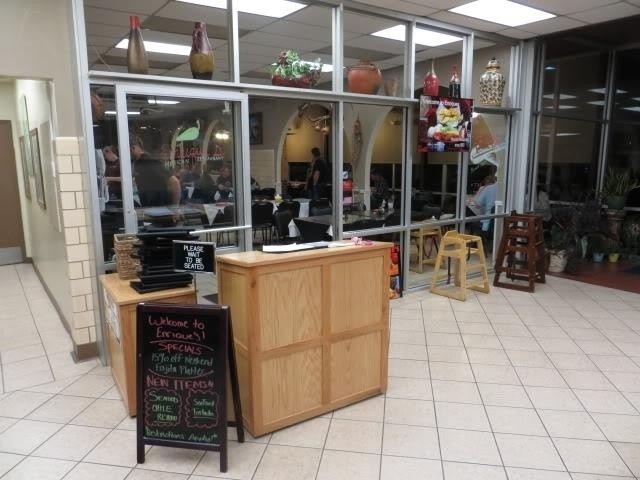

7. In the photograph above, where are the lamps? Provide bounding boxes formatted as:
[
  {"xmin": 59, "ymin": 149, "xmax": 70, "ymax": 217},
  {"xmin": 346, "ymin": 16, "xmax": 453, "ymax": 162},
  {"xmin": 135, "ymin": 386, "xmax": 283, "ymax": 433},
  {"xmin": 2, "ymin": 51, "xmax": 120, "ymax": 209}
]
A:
[{"xmin": 213, "ymin": 128, "xmax": 231, "ymax": 142}]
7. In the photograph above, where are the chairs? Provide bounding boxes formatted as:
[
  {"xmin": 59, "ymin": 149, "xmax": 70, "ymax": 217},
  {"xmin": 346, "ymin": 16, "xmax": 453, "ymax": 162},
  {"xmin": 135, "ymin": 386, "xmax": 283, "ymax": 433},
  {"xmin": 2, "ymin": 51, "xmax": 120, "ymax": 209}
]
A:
[
  {"xmin": 263, "ymin": 188, "xmax": 275, "ymax": 200},
  {"xmin": 271, "ymin": 209, "xmax": 296, "ymax": 242},
  {"xmin": 413, "ymin": 205, "xmax": 442, "ymax": 259},
  {"xmin": 308, "ymin": 197, "xmax": 332, "ymax": 216},
  {"xmin": 410, "ymin": 226, "xmax": 449, "ymax": 273},
  {"xmin": 252, "ymin": 201, "xmax": 275, "ymax": 243},
  {"xmin": 273, "ymin": 201, "xmax": 301, "ymax": 242},
  {"xmin": 201, "ymin": 208, "xmax": 223, "ymax": 243},
  {"xmin": 386, "ymin": 212, "xmax": 400, "ymax": 242},
  {"xmin": 349, "ymin": 219, "xmax": 379, "ymax": 241},
  {"xmin": 218, "ymin": 205, "xmax": 237, "ymax": 247}
]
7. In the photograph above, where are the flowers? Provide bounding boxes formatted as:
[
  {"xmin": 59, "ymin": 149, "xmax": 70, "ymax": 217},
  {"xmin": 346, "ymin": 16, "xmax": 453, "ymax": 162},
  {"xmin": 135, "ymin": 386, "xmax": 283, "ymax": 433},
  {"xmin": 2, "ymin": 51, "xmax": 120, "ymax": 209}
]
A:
[{"xmin": 544, "ymin": 221, "xmax": 575, "ymax": 253}]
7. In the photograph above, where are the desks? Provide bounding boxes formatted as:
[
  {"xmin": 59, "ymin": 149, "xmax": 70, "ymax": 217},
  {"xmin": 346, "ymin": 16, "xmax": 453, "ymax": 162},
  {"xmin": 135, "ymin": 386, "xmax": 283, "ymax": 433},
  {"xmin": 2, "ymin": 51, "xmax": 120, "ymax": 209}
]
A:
[
  {"xmin": 292, "ymin": 209, "xmax": 387, "ymax": 243},
  {"xmin": 100, "ymin": 269, "xmax": 198, "ymax": 417},
  {"xmin": 215, "ymin": 239, "xmax": 394, "ymax": 438}
]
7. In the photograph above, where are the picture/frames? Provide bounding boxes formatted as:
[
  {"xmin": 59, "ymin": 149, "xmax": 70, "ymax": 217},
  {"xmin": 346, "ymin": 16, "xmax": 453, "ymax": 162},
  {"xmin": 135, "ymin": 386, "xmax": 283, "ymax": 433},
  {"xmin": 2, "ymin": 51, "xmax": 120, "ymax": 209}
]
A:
[
  {"xmin": 18, "ymin": 135, "xmax": 33, "ymax": 203},
  {"xmin": 28, "ymin": 125, "xmax": 47, "ymax": 211},
  {"xmin": 249, "ymin": 112, "xmax": 264, "ymax": 146}
]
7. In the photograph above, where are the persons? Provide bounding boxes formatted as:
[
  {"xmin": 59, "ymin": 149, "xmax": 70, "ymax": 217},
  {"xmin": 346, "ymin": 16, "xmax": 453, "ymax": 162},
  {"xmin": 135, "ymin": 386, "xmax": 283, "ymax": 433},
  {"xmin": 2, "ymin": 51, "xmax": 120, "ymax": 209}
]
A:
[
  {"xmin": 100, "ymin": 144, "xmax": 121, "ymax": 199},
  {"xmin": 189, "ymin": 160, "xmax": 215, "ymax": 204},
  {"xmin": 128, "ymin": 133, "xmax": 183, "ymax": 226},
  {"xmin": 476, "ymin": 171, "xmax": 498, "ymax": 232},
  {"xmin": 307, "ymin": 147, "xmax": 327, "ymax": 202},
  {"xmin": 475, "ymin": 176, "xmax": 493, "ymax": 209},
  {"xmin": 216, "ymin": 164, "xmax": 233, "ymax": 200},
  {"xmin": 371, "ymin": 168, "xmax": 392, "ymax": 208},
  {"xmin": 536, "ymin": 178, "xmax": 552, "ymax": 246}
]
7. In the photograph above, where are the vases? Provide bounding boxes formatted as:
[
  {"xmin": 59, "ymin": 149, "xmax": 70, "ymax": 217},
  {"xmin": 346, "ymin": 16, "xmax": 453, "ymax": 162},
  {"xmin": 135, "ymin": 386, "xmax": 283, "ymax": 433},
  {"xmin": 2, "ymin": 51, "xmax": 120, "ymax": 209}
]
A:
[
  {"xmin": 345, "ymin": 60, "xmax": 384, "ymax": 96},
  {"xmin": 422, "ymin": 57, "xmax": 441, "ymax": 96},
  {"xmin": 478, "ymin": 55, "xmax": 508, "ymax": 107},
  {"xmin": 126, "ymin": 14, "xmax": 149, "ymax": 76},
  {"xmin": 188, "ymin": 20, "xmax": 215, "ymax": 82},
  {"xmin": 543, "ymin": 246, "xmax": 572, "ymax": 278},
  {"xmin": 449, "ymin": 64, "xmax": 462, "ymax": 98}
]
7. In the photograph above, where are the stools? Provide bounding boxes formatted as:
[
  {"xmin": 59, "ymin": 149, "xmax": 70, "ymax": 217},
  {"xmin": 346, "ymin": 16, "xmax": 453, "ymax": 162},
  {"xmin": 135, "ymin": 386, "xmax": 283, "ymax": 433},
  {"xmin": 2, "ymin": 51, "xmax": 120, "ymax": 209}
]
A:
[
  {"xmin": 492, "ymin": 213, "xmax": 542, "ymax": 292},
  {"xmin": 429, "ymin": 230, "xmax": 491, "ymax": 302}
]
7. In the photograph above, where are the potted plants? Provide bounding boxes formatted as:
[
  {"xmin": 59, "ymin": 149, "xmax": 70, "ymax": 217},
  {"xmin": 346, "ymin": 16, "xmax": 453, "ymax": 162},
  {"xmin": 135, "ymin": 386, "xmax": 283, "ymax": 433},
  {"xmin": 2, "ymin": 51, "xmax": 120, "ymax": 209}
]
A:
[
  {"xmin": 270, "ymin": 46, "xmax": 325, "ymax": 89},
  {"xmin": 573, "ymin": 166, "xmax": 640, "ymax": 264}
]
